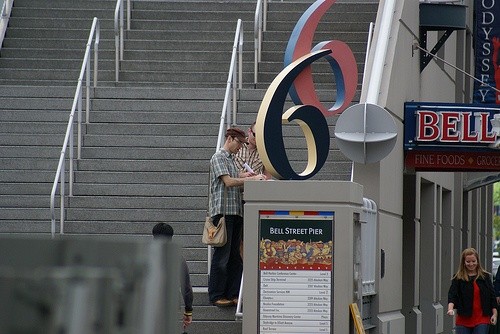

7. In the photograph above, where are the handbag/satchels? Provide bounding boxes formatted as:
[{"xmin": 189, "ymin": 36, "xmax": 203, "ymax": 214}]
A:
[{"xmin": 202, "ymin": 217, "xmax": 228, "ymax": 248}]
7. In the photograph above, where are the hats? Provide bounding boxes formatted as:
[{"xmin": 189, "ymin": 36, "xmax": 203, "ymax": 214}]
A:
[{"xmin": 227, "ymin": 128, "xmax": 250, "ymax": 145}]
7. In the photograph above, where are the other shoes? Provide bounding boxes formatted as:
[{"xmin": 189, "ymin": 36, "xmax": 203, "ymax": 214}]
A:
[
  {"xmin": 209, "ymin": 297, "xmax": 234, "ymax": 308},
  {"xmin": 230, "ymin": 297, "xmax": 243, "ymax": 305}
]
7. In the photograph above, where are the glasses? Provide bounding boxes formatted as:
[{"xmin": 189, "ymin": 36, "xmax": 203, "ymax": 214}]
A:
[
  {"xmin": 230, "ymin": 136, "xmax": 245, "ymax": 148},
  {"xmin": 250, "ymin": 128, "xmax": 256, "ymax": 138}
]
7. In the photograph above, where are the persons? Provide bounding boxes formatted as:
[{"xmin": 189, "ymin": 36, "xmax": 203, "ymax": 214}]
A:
[
  {"xmin": 152, "ymin": 223, "xmax": 193, "ymax": 328},
  {"xmin": 208, "ymin": 128, "xmax": 267, "ymax": 306},
  {"xmin": 232, "ymin": 121, "xmax": 270, "ymax": 261},
  {"xmin": 447, "ymin": 248, "xmax": 500, "ymax": 334}
]
